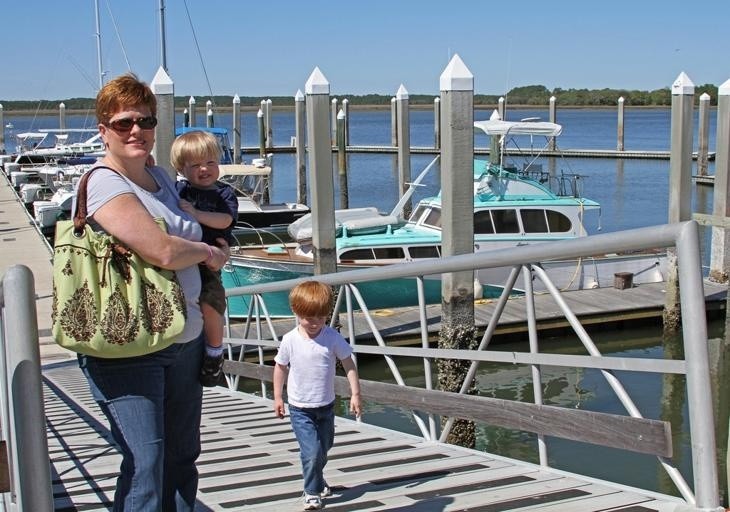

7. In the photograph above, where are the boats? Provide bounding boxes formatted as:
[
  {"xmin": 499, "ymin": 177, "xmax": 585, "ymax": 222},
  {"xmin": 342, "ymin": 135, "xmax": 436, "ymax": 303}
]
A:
[{"xmin": 213, "ymin": 115, "xmax": 681, "ymax": 321}]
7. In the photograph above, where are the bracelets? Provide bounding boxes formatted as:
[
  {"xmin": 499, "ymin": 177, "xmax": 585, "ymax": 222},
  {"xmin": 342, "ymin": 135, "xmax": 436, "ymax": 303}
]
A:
[{"xmin": 198, "ymin": 241, "xmax": 213, "ymax": 267}]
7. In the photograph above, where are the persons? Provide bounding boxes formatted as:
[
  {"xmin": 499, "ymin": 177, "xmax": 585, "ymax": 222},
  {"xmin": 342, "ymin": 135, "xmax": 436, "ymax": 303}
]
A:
[
  {"xmin": 71, "ymin": 73, "xmax": 230, "ymax": 512},
  {"xmin": 273, "ymin": 280, "xmax": 361, "ymax": 509},
  {"xmin": 146, "ymin": 131, "xmax": 239, "ymax": 387}
]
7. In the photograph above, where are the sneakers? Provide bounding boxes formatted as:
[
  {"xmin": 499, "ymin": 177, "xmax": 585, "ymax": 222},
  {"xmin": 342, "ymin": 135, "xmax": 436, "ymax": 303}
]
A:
[
  {"xmin": 199, "ymin": 348, "xmax": 224, "ymax": 388},
  {"xmin": 319, "ymin": 478, "xmax": 332, "ymax": 497},
  {"xmin": 302, "ymin": 492, "xmax": 323, "ymax": 512}
]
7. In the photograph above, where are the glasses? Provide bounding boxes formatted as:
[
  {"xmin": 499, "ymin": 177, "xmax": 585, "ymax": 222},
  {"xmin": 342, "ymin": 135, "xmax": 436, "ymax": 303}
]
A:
[{"xmin": 105, "ymin": 116, "xmax": 158, "ymax": 132}]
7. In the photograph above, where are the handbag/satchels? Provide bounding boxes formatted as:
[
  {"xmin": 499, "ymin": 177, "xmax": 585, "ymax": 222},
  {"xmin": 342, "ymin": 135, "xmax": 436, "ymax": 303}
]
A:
[{"xmin": 50, "ymin": 166, "xmax": 189, "ymax": 361}]
